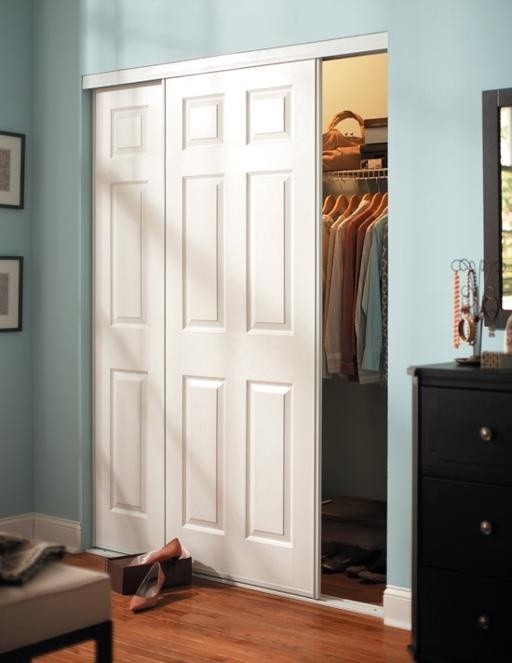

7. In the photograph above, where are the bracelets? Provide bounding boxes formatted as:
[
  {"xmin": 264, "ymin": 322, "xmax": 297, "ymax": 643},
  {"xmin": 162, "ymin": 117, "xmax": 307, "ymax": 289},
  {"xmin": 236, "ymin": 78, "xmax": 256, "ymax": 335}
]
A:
[{"xmin": 458, "ymin": 316, "xmax": 475, "ymax": 342}]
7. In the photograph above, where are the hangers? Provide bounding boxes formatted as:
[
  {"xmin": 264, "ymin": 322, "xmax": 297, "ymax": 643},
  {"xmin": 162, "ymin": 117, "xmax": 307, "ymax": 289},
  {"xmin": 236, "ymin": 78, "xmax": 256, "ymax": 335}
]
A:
[{"xmin": 320, "ymin": 170, "xmax": 390, "ymax": 230}]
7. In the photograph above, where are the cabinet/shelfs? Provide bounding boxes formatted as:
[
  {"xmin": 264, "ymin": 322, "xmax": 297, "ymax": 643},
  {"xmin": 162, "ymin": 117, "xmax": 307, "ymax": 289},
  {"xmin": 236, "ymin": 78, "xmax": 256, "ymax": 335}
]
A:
[{"xmin": 409, "ymin": 364, "xmax": 510, "ymax": 662}]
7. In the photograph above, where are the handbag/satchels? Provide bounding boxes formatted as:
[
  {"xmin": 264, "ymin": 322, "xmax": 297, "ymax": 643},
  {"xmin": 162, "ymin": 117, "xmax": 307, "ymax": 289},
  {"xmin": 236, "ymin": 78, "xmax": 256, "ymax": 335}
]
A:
[{"xmin": 322, "ymin": 110, "xmax": 365, "ymax": 172}]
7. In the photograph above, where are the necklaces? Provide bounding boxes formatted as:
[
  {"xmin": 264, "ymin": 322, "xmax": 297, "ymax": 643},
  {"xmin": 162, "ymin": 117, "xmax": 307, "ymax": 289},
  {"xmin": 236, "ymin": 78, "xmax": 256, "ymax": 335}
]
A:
[
  {"xmin": 466, "ymin": 270, "xmax": 479, "ymax": 323},
  {"xmin": 453, "ymin": 271, "xmax": 461, "ymax": 348}
]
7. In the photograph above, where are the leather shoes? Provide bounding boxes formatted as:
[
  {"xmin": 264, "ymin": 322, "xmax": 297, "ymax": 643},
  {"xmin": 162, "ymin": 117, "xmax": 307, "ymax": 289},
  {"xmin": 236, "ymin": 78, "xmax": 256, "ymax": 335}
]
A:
[{"xmin": 321, "ymin": 541, "xmax": 385, "ymax": 583}]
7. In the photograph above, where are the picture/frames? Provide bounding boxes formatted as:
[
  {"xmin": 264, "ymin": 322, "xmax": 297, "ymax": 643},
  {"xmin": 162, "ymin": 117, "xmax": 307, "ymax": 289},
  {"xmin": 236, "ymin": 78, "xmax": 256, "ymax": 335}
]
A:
[
  {"xmin": 0, "ymin": 257, "xmax": 26, "ymax": 335},
  {"xmin": 0, "ymin": 129, "xmax": 28, "ymax": 210},
  {"xmin": 478, "ymin": 88, "xmax": 512, "ymax": 335}
]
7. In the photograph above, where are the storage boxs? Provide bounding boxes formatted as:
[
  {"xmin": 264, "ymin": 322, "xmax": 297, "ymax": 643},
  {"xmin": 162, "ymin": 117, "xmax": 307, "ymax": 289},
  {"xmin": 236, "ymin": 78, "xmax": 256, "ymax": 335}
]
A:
[{"xmin": 355, "ymin": 116, "xmax": 387, "ymax": 170}]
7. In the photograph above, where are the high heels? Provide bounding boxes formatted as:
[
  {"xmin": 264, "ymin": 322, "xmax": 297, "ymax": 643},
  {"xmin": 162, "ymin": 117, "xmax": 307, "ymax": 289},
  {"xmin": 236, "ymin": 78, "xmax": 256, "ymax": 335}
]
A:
[
  {"xmin": 146, "ymin": 537, "xmax": 182, "ymax": 569},
  {"xmin": 130, "ymin": 561, "xmax": 166, "ymax": 610}
]
